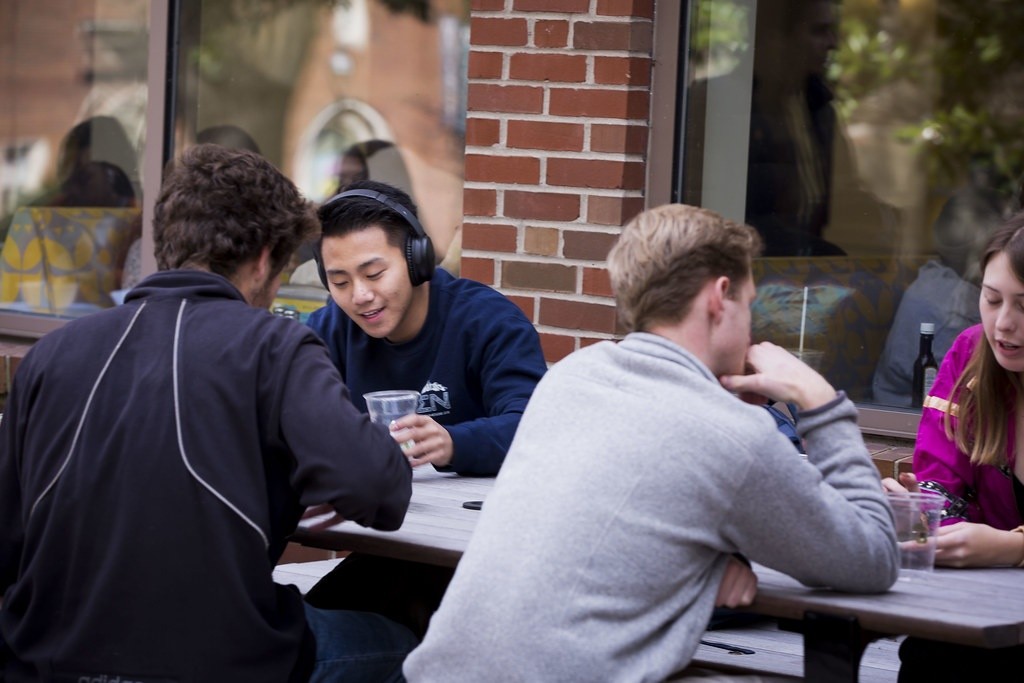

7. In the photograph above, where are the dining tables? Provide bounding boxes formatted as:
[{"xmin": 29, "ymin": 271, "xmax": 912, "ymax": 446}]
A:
[{"xmin": 283, "ymin": 459, "xmax": 1023, "ymax": 683}]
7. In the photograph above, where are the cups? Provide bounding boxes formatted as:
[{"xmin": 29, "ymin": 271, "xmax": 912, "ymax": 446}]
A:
[
  {"xmin": 362, "ymin": 390, "xmax": 422, "ymax": 466},
  {"xmin": 886, "ymin": 492, "xmax": 947, "ymax": 583}
]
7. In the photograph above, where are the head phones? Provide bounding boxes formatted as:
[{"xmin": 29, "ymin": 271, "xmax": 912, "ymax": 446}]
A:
[{"xmin": 311, "ymin": 188, "xmax": 435, "ymax": 293}]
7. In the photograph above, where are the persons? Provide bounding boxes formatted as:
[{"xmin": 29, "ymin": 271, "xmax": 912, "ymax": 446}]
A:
[
  {"xmin": 0, "ymin": 139, "xmax": 413, "ymax": 683},
  {"xmin": 395, "ymin": 203, "xmax": 904, "ymax": 681},
  {"xmin": 870, "ymin": 200, "xmax": 1005, "ymax": 406},
  {"xmin": 280, "ymin": 179, "xmax": 549, "ymax": 626},
  {"xmin": 892, "ymin": 207, "xmax": 1024, "ymax": 683},
  {"xmin": 38, "ymin": 115, "xmax": 405, "ymax": 209}
]
